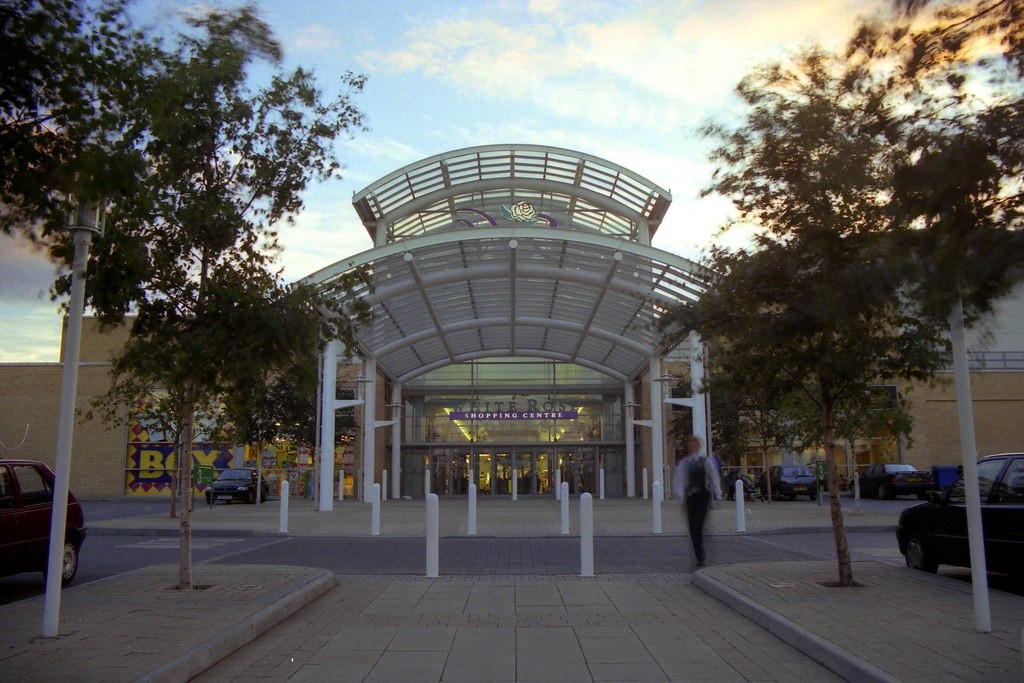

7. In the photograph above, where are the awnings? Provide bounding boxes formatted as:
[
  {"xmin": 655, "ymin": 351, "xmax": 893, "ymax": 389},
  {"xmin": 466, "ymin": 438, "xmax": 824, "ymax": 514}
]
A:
[
  {"xmin": 864, "ymin": 385, "xmax": 899, "ymax": 411},
  {"xmin": 671, "ymin": 387, "xmax": 761, "ymax": 411}
]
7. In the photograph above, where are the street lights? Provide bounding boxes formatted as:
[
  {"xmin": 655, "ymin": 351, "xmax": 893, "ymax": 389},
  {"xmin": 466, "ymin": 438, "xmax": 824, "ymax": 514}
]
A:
[{"xmin": 41, "ymin": 180, "xmax": 114, "ymax": 640}]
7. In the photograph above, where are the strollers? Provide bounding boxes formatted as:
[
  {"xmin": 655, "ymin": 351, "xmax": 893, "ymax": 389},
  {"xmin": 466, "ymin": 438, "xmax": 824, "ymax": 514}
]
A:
[{"xmin": 736, "ymin": 474, "xmax": 765, "ymax": 502}]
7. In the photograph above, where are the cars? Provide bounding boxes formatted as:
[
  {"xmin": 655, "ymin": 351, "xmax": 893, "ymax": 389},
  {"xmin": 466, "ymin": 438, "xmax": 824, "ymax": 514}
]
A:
[
  {"xmin": 758, "ymin": 465, "xmax": 818, "ymax": 501},
  {"xmin": 206, "ymin": 469, "xmax": 268, "ymax": 505},
  {"xmin": 849, "ymin": 462, "xmax": 935, "ymax": 500},
  {"xmin": 895, "ymin": 451, "xmax": 1024, "ymax": 575},
  {"xmin": 0, "ymin": 459, "xmax": 87, "ymax": 588}
]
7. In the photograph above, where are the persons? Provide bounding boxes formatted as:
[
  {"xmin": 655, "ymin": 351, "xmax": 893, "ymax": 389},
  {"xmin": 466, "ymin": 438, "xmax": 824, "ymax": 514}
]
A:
[
  {"xmin": 726, "ymin": 469, "xmax": 739, "ymax": 501},
  {"xmin": 748, "ymin": 468, "xmax": 768, "ymax": 503},
  {"xmin": 712, "ymin": 447, "xmax": 722, "ymax": 502},
  {"xmin": 671, "ymin": 436, "xmax": 722, "ymax": 566}
]
